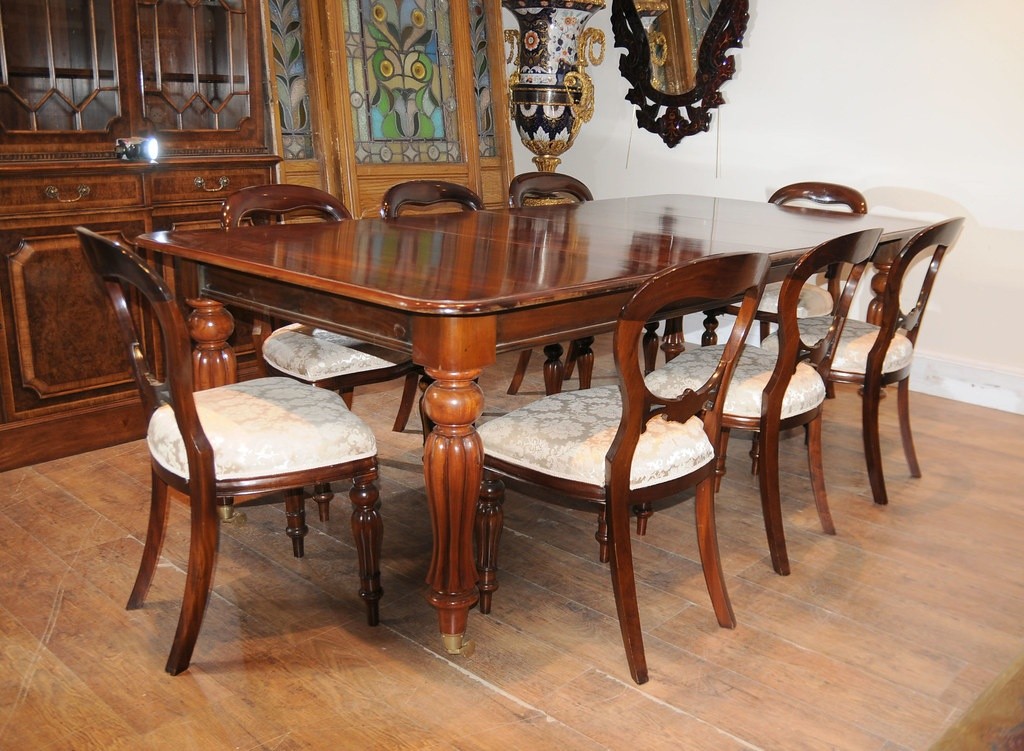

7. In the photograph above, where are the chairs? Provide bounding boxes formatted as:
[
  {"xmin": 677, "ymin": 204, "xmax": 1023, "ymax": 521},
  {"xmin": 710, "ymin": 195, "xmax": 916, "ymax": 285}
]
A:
[
  {"xmin": 382, "ymin": 180, "xmax": 563, "ymax": 458},
  {"xmin": 701, "ymin": 182, "xmax": 866, "ymax": 447},
  {"xmin": 713, "ymin": 215, "xmax": 967, "ymax": 503},
  {"xmin": 220, "ymin": 183, "xmax": 434, "ymax": 522},
  {"xmin": 509, "ymin": 172, "xmax": 659, "ymax": 389},
  {"xmin": 471, "ymin": 251, "xmax": 772, "ymax": 686},
  {"xmin": 636, "ymin": 227, "xmax": 883, "ymax": 575},
  {"xmin": 75, "ymin": 226, "xmax": 383, "ymax": 676}
]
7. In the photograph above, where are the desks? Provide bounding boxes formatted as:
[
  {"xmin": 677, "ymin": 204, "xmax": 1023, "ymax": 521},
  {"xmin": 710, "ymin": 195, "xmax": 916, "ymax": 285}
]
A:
[{"xmin": 136, "ymin": 195, "xmax": 945, "ymax": 614}]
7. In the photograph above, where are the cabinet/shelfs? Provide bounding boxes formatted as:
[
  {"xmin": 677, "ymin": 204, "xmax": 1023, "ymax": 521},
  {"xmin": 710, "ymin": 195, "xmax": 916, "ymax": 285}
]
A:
[{"xmin": 0, "ymin": 1, "xmax": 290, "ymax": 473}]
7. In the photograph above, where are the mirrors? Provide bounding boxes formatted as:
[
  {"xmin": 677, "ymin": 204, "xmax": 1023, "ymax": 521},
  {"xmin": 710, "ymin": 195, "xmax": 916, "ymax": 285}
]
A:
[{"xmin": 609, "ymin": 0, "xmax": 751, "ymax": 148}]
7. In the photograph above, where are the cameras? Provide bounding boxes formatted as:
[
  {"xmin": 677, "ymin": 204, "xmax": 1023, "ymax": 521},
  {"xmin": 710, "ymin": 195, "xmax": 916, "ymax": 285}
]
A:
[{"xmin": 114, "ymin": 138, "xmax": 157, "ymax": 161}]
